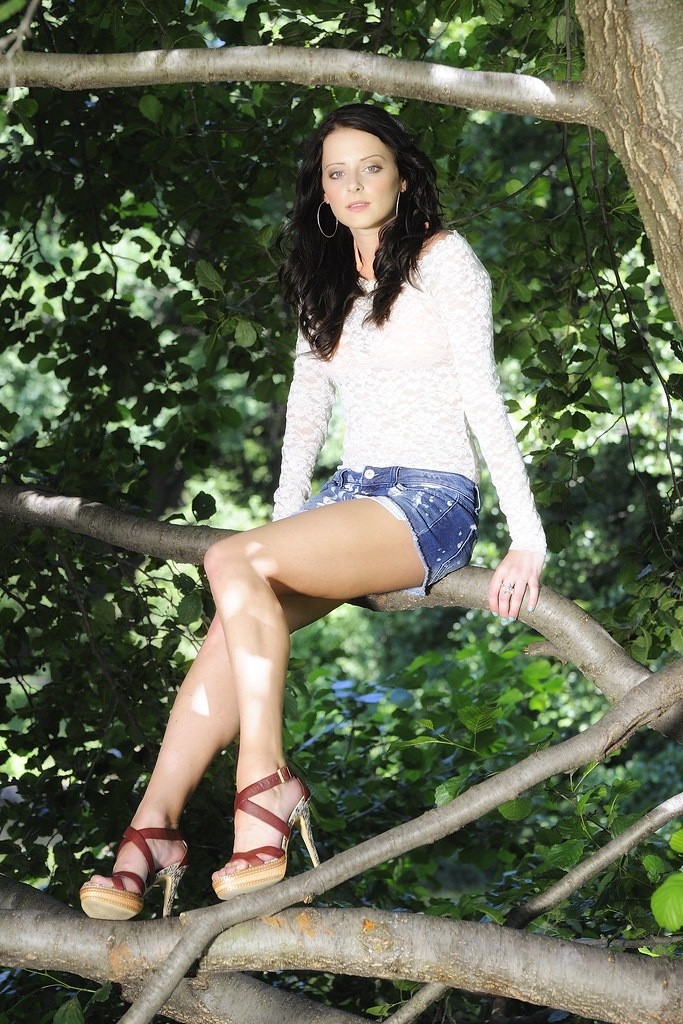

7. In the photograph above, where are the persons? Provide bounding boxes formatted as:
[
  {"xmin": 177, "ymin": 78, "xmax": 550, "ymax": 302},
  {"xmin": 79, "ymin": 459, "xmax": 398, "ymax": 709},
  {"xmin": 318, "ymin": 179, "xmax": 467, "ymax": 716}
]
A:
[{"xmin": 80, "ymin": 104, "xmax": 547, "ymax": 922}]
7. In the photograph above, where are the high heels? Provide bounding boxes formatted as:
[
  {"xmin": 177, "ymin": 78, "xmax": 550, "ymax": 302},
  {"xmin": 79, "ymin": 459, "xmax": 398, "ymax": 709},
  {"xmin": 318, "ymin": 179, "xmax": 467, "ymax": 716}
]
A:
[
  {"xmin": 211, "ymin": 765, "xmax": 320, "ymax": 900},
  {"xmin": 79, "ymin": 826, "xmax": 191, "ymax": 921}
]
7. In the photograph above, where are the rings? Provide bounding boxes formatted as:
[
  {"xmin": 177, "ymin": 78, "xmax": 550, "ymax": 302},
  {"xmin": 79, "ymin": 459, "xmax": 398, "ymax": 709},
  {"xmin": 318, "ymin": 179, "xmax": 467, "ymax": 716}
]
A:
[{"xmin": 501, "ymin": 583, "xmax": 515, "ymax": 594}]
